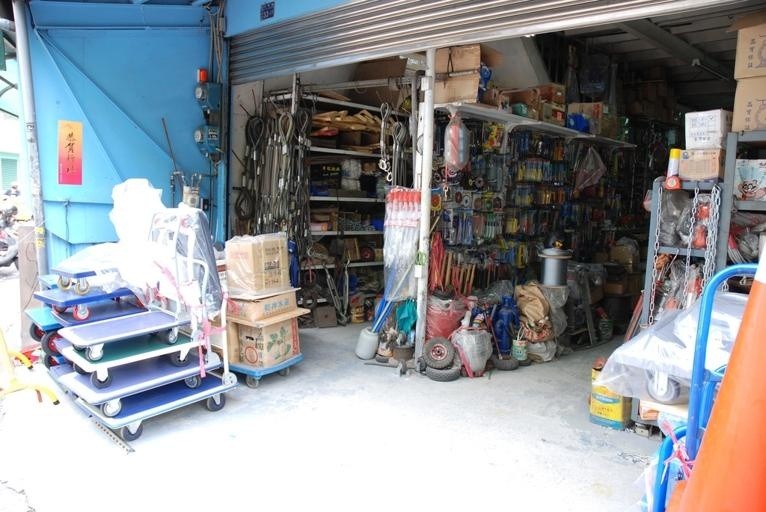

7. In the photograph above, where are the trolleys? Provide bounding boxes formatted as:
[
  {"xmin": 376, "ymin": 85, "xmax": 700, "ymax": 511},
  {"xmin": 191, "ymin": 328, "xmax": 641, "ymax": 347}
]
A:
[
  {"xmin": 21, "ymin": 206, "xmax": 239, "ymax": 441},
  {"xmin": 195, "ymin": 251, "xmax": 303, "ymax": 389},
  {"xmin": 609, "ymin": 288, "xmax": 749, "ymax": 409}
]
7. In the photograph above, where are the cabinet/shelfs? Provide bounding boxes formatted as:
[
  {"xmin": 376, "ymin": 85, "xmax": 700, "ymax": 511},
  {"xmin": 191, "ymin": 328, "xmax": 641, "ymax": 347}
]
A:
[
  {"xmin": 637, "ymin": 130, "xmax": 764, "ymax": 337},
  {"xmin": 260, "ymin": 91, "xmax": 412, "ymax": 326}
]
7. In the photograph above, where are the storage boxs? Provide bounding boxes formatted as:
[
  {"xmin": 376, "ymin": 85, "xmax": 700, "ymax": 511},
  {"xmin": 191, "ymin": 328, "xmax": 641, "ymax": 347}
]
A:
[
  {"xmin": 673, "ymin": 23, "xmax": 765, "ymax": 181},
  {"xmin": 223, "ymin": 233, "xmax": 312, "ymax": 370}
]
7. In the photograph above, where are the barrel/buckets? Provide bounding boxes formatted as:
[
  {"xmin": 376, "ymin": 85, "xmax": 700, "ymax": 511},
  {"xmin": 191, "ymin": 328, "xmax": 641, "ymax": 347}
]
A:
[{"xmin": 537, "ymin": 240, "xmax": 573, "ymax": 287}]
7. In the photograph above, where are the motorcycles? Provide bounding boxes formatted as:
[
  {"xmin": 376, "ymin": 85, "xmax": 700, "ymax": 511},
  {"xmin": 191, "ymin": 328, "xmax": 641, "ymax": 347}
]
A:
[{"xmin": 0, "ymin": 201, "xmax": 22, "ymax": 271}]
